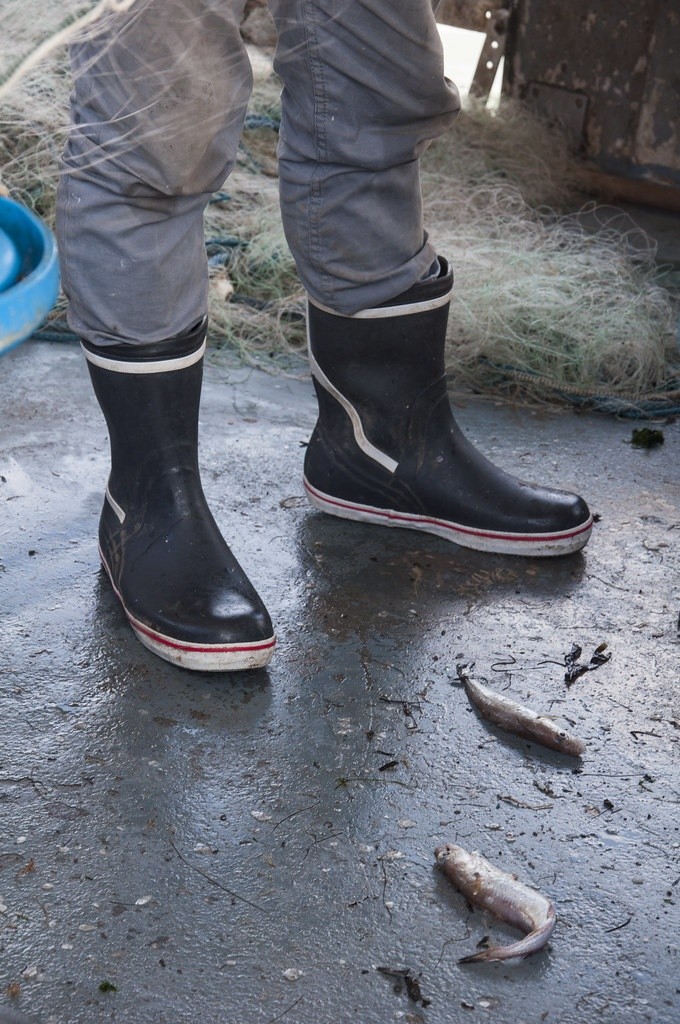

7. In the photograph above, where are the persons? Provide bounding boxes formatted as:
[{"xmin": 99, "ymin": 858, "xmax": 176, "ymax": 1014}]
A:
[{"xmin": 52, "ymin": 0, "xmax": 596, "ymax": 676}]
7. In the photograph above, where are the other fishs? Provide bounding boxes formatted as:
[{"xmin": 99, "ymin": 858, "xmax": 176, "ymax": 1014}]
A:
[
  {"xmin": 455, "ymin": 664, "xmax": 584, "ymax": 759},
  {"xmin": 434, "ymin": 842, "xmax": 557, "ymax": 964}
]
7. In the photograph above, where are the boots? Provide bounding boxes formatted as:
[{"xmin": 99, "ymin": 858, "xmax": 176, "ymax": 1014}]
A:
[
  {"xmin": 79, "ymin": 312, "xmax": 276, "ymax": 670},
  {"xmin": 302, "ymin": 254, "xmax": 593, "ymax": 559}
]
